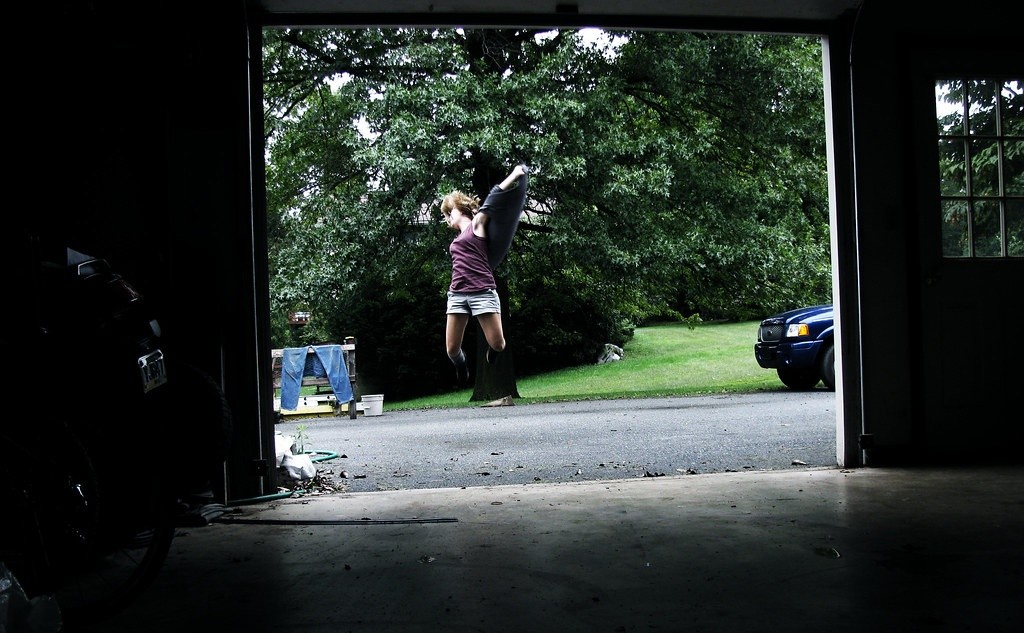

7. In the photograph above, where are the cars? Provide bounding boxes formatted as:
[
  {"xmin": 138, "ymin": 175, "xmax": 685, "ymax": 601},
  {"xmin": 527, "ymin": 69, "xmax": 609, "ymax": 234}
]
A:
[{"xmin": 754, "ymin": 305, "xmax": 835, "ymax": 390}]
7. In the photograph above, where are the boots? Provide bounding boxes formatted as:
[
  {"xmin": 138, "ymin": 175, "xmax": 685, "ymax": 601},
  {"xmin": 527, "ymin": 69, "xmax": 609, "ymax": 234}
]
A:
[
  {"xmin": 486, "ymin": 346, "xmax": 497, "ymax": 364},
  {"xmin": 449, "ymin": 349, "xmax": 469, "ymax": 382}
]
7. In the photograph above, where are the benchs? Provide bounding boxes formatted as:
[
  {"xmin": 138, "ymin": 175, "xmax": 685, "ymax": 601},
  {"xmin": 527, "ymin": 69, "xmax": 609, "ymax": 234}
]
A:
[{"xmin": 271, "ymin": 335, "xmax": 357, "ymax": 420}]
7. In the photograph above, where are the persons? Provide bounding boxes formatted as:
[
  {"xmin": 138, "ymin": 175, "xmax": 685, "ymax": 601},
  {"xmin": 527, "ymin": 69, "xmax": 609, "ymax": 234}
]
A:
[{"xmin": 438, "ymin": 164, "xmax": 529, "ymax": 382}]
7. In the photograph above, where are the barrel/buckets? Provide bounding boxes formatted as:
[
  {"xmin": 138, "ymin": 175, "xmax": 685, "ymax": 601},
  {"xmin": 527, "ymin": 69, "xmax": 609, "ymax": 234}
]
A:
[{"xmin": 361, "ymin": 395, "xmax": 384, "ymax": 416}]
